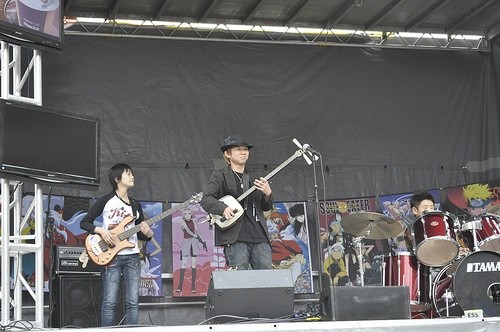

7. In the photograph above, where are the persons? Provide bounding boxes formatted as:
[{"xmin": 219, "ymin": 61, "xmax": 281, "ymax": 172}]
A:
[
  {"xmin": 410, "ymin": 191, "xmax": 435, "ymax": 303},
  {"xmin": 80, "ymin": 163, "xmax": 154, "ymax": 327},
  {"xmin": 201, "ymin": 134, "xmax": 273, "ymax": 270}
]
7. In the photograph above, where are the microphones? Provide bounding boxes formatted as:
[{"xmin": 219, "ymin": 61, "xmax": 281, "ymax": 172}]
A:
[{"xmin": 303, "ymin": 143, "xmax": 321, "ymax": 155}]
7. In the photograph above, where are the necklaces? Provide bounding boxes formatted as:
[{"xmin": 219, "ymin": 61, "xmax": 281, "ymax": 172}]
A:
[{"xmin": 232, "ymin": 169, "xmax": 243, "ymax": 188}]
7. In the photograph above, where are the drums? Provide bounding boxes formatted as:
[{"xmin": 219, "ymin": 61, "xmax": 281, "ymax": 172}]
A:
[
  {"xmin": 413, "ymin": 210, "xmax": 460, "ymax": 267},
  {"xmin": 470, "ymin": 213, "xmax": 500, "ymax": 252},
  {"xmin": 432, "ymin": 250, "xmax": 500, "ymax": 318},
  {"xmin": 379, "ymin": 251, "xmax": 432, "ymax": 312}
]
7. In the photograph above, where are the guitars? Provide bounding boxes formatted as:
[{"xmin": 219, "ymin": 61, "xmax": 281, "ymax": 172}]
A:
[{"xmin": 85, "ymin": 192, "xmax": 203, "ymax": 267}]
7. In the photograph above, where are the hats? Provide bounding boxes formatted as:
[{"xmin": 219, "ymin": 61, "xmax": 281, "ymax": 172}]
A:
[{"xmin": 220, "ymin": 137, "xmax": 253, "ymax": 153}]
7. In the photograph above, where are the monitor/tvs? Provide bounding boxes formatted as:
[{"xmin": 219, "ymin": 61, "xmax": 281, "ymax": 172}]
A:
[{"xmin": 0, "ymin": 98, "xmax": 101, "ymax": 187}]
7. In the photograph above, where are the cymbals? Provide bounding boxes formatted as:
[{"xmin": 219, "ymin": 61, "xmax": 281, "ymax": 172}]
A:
[{"xmin": 340, "ymin": 212, "xmax": 403, "ymax": 239}]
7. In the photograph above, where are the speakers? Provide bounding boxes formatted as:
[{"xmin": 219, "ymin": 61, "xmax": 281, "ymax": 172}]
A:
[
  {"xmin": 46, "ymin": 275, "xmax": 127, "ymax": 327},
  {"xmin": 320, "ymin": 285, "xmax": 414, "ymax": 321},
  {"xmin": 202, "ymin": 268, "xmax": 296, "ymax": 325}
]
